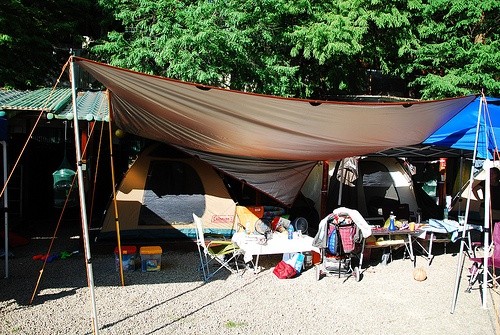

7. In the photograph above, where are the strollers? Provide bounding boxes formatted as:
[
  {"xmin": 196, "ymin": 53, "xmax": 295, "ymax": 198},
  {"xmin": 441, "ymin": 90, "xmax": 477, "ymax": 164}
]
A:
[{"xmin": 315, "ymin": 208, "xmax": 372, "ymax": 282}]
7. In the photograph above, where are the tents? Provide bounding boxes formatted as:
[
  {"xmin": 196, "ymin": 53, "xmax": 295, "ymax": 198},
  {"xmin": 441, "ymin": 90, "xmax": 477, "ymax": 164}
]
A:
[{"xmin": 0, "ymin": 51, "xmax": 500, "ymax": 335}]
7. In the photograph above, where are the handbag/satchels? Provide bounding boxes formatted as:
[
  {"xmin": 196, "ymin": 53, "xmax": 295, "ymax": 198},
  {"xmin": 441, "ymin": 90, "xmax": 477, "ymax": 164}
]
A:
[
  {"xmin": 273, "ymin": 260, "xmax": 297, "ymax": 279},
  {"xmin": 286, "ymin": 250, "xmax": 305, "ymax": 273}
]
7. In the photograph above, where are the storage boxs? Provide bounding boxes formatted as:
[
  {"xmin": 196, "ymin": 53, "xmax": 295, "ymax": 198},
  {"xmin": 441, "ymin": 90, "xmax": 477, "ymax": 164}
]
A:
[
  {"xmin": 114, "ymin": 246, "xmax": 136, "ymax": 272},
  {"xmin": 140, "ymin": 246, "xmax": 162, "ymax": 273}
]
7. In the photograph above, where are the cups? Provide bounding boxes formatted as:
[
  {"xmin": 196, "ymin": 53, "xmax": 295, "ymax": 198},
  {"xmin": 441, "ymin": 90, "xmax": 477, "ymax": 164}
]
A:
[
  {"xmin": 410, "ymin": 223, "xmax": 415, "ymax": 231},
  {"xmin": 458, "ymin": 216, "xmax": 464, "ymax": 226}
]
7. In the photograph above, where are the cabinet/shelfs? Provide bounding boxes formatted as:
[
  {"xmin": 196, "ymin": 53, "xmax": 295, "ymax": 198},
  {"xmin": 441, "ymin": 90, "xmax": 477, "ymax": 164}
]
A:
[{"xmin": 7, "ymin": 164, "xmax": 23, "ymax": 216}]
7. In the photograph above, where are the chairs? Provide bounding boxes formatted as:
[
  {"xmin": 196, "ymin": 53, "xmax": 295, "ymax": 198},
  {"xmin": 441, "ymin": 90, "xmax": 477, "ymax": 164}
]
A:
[
  {"xmin": 465, "ymin": 222, "xmax": 500, "ymax": 294},
  {"xmin": 192, "ymin": 213, "xmax": 243, "ymax": 283}
]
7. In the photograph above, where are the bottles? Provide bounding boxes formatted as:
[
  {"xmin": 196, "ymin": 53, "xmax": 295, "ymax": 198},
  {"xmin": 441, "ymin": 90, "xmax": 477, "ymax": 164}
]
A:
[
  {"xmin": 288, "ymin": 225, "xmax": 293, "ymax": 239},
  {"xmin": 388, "ymin": 211, "xmax": 395, "ymax": 230}
]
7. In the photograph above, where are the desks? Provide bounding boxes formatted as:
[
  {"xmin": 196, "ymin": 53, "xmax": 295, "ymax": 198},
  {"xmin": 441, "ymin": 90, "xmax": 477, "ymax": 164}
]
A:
[
  {"xmin": 232, "ymin": 235, "xmax": 321, "ymax": 275},
  {"xmin": 364, "ymin": 229, "xmax": 422, "ymax": 267},
  {"xmin": 410, "ymin": 222, "xmax": 484, "ymax": 266}
]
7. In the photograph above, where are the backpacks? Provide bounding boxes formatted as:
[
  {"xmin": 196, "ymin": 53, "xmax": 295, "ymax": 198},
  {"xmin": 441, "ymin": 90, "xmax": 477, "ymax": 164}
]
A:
[{"xmin": 337, "ymin": 157, "xmax": 358, "ymax": 187}]
